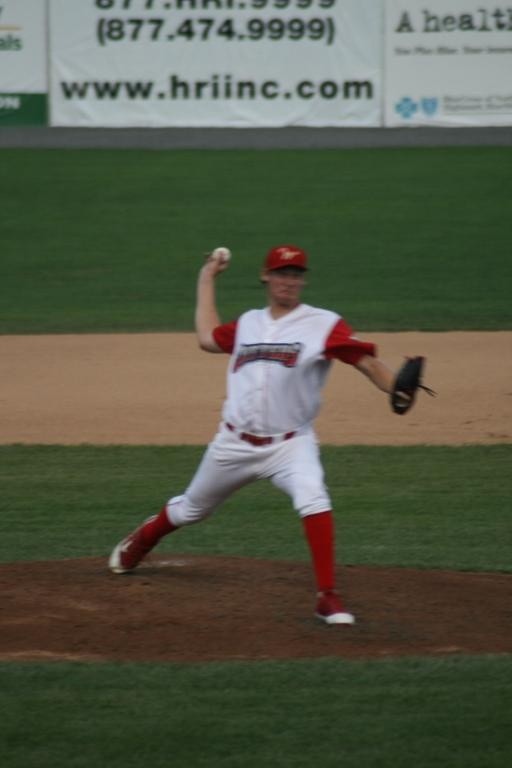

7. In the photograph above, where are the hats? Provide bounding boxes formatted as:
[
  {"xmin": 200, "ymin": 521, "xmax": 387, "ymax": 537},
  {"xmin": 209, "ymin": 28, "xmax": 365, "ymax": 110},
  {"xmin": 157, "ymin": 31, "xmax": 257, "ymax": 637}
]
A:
[{"xmin": 266, "ymin": 244, "xmax": 308, "ymax": 273}]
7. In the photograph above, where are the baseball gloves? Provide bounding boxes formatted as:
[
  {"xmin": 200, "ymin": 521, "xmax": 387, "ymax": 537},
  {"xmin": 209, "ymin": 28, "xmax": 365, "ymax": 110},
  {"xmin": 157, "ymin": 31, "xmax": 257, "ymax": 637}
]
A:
[{"xmin": 391, "ymin": 357, "xmax": 438, "ymax": 415}]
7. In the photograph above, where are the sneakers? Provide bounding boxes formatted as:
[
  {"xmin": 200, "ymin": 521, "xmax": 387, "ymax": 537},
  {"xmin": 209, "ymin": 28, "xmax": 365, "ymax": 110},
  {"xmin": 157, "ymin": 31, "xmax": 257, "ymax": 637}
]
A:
[
  {"xmin": 314, "ymin": 588, "xmax": 355, "ymax": 625},
  {"xmin": 108, "ymin": 513, "xmax": 160, "ymax": 573}
]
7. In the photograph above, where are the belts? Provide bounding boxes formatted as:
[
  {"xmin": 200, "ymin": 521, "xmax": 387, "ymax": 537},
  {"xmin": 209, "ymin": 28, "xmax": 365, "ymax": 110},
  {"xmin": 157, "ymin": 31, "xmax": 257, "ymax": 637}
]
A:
[{"xmin": 225, "ymin": 422, "xmax": 296, "ymax": 447}]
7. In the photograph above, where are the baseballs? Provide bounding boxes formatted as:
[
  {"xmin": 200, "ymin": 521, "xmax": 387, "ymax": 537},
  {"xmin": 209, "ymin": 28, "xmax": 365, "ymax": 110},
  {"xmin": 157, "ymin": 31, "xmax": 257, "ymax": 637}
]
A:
[{"xmin": 212, "ymin": 247, "xmax": 231, "ymax": 263}]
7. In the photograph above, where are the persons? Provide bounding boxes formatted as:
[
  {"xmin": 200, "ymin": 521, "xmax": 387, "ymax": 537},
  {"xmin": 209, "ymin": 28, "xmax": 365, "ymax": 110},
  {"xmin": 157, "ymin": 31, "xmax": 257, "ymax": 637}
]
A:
[{"xmin": 104, "ymin": 245, "xmax": 426, "ymax": 627}]
